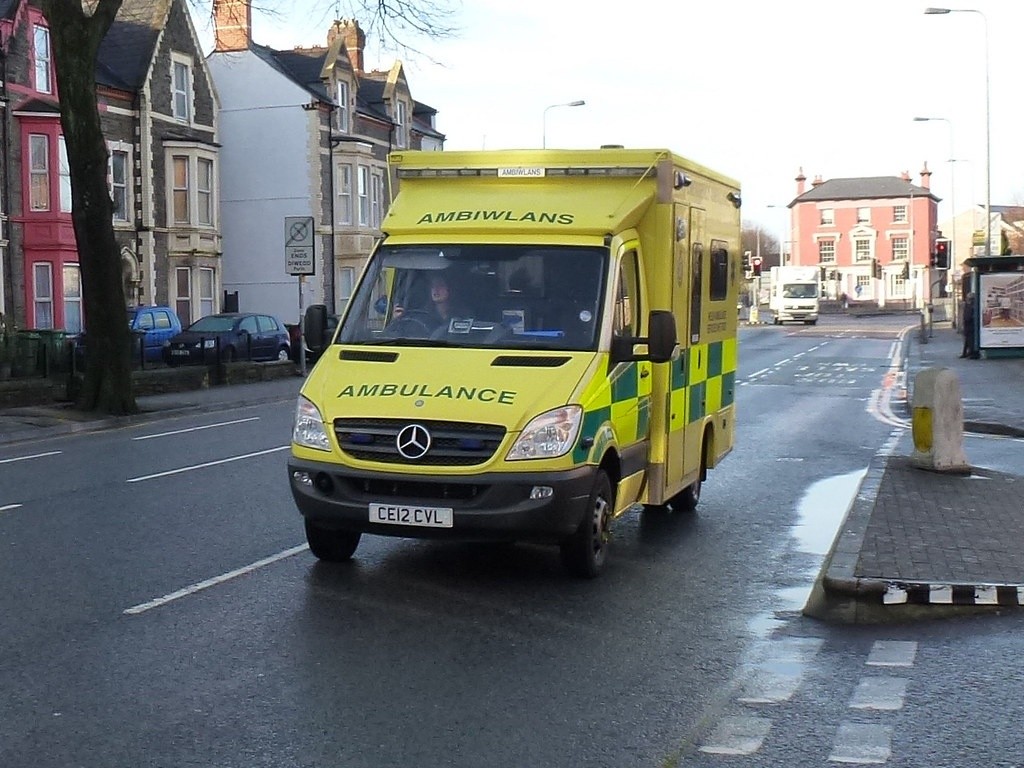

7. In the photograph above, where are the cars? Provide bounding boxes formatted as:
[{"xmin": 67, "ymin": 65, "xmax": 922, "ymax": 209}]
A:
[
  {"xmin": 126, "ymin": 306, "xmax": 182, "ymax": 361},
  {"xmin": 161, "ymin": 313, "xmax": 291, "ymax": 367}
]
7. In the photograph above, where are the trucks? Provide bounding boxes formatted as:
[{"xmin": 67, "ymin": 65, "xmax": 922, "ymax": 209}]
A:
[{"xmin": 770, "ymin": 265, "xmax": 820, "ymax": 326}]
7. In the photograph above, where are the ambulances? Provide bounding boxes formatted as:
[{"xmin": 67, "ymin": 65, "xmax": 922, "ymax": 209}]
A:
[{"xmin": 286, "ymin": 148, "xmax": 742, "ymax": 576}]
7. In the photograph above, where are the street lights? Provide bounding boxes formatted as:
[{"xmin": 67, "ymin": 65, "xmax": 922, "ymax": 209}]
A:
[
  {"xmin": 766, "ymin": 205, "xmax": 783, "ymax": 267},
  {"xmin": 923, "ymin": 6, "xmax": 992, "ymax": 256},
  {"xmin": 914, "ymin": 116, "xmax": 958, "ymax": 329},
  {"xmin": 946, "ymin": 158, "xmax": 975, "ymax": 229},
  {"xmin": 543, "ymin": 100, "xmax": 586, "ymax": 148}
]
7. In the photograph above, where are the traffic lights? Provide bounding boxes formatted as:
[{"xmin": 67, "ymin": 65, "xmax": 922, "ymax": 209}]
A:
[
  {"xmin": 741, "ymin": 250, "xmax": 752, "ymax": 279},
  {"xmin": 751, "ymin": 257, "xmax": 762, "ymax": 278}
]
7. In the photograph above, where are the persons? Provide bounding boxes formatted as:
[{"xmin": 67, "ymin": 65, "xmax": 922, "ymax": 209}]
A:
[
  {"xmin": 999, "ymin": 294, "xmax": 1011, "ymax": 321},
  {"xmin": 388, "ymin": 274, "xmax": 459, "ymax": 338}
]
